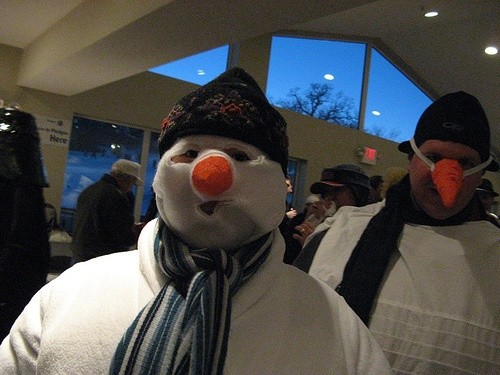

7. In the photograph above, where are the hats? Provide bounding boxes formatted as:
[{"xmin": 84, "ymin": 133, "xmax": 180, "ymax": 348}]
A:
[
  {"xmin": 310, "ymin": 164, "xmax": 371, "ymax": 206},
  {"xmin": 112, "ymin": 159, "xmax": 145, "ymax": 186},
  {"xmin": 398, "ymin": 90, "xmax": 499, "ymax": 172},
  {"xmin": 158, "ymin": 67, "xmax": 289, "ymax": 177},
  {"xmin": 475, "ymin": 179, "xmax": 499, "ymax": 197}
]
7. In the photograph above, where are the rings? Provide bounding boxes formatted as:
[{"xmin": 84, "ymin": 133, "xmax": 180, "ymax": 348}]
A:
[{"xmin": 300, "ymin": 229, "xmax": 306, "ymax": 233}]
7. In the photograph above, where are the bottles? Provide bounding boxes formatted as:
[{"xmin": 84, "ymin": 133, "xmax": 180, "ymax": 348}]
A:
[{"xmin": 303, "ymin": 202, "xmax": 335, "ymax": 228}]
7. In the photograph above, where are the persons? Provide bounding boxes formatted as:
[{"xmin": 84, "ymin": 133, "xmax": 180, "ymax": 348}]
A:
[
  {"xmin": 0, "ymin": 67, "xmax": 393, "ymax": 375},
  {"xmin": 307, "ymin": 91, "xmax": 500, "ymax": 375},
  {"xmin": 70, "ymin": 159, "xmax": 500, "ymax": 265}
]
